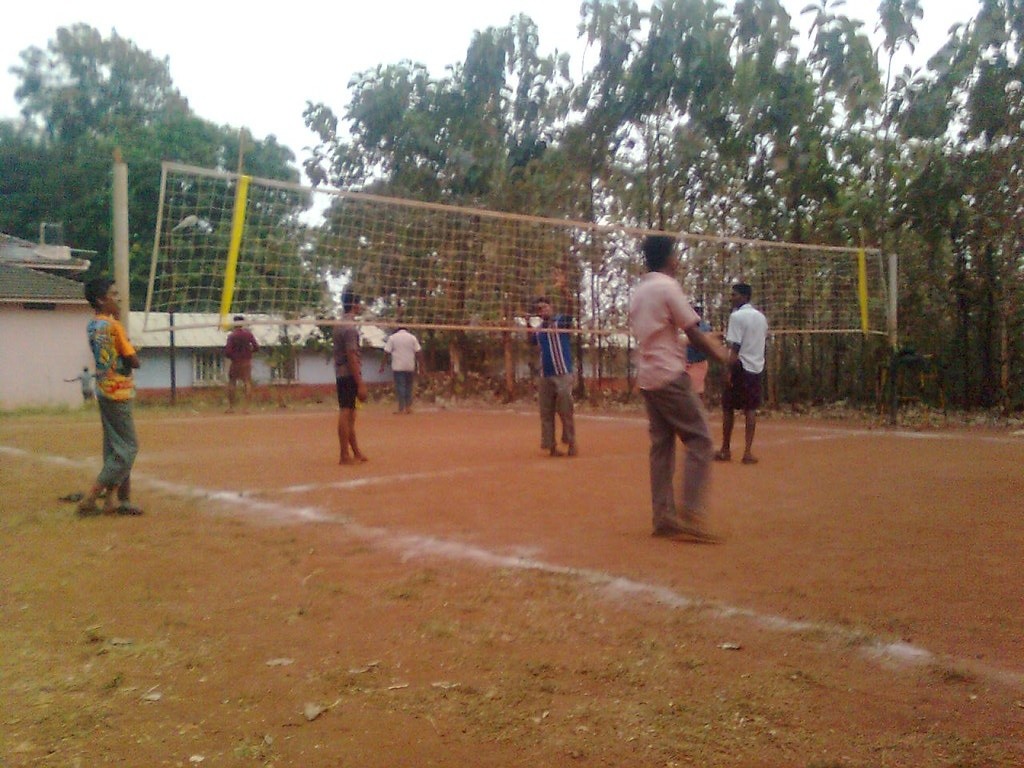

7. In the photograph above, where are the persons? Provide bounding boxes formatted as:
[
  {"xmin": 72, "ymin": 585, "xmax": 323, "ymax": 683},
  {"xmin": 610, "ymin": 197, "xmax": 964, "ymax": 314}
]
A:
[
  {"xmin": 684, "ymin": 301, "xmax": 712, "ymax": 397},
  {"xmin": 713, "ymin": 280, "xmax": 766, "ymax": 462},
  {"xmin": 75, "ymin": 277, "xmax": 146, "ymax": 516},
  {"xmin": 628, "ymin": 233, "xmax": 735, "ymax": 542},
  {"xmin": 65, "ymin": 367, "xmax": 96, "ymax": 399},
  {"xmin": 330, "ymin": 288, "xmax": 370, "ymax": 465},
  {"xmin": 522, "ymin": 297, "xmax": 578, "ymax": 456},
  {"xmin": 379, "ymin": 320, "xmax": 431, "ymax": 415},
  {"xmin": 224, "ymin": 315, "xmax": 259, "ymax": 415}
]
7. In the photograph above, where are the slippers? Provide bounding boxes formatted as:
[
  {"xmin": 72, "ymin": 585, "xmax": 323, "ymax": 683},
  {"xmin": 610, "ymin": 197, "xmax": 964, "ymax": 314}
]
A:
[
  {"xmin": 103, "ymin": 502, "xmax": 143, "ymax": 516},
  {"xmin": 74, "ymin": 501, "xmax": 102, "ymax": 515}
]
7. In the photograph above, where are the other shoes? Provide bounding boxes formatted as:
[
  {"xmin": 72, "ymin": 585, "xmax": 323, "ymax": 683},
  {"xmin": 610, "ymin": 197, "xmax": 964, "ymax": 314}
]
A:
[
  {"xmin": 650, "ymin": 518, "xmax": 673, "ymax": 538},
  {"xmin": 667, "ymin": 516, "xmax": 724, "ymax": 543},
  {"xmin": 550, "ymin": 450, "xmax": 565, "ymax": 457},
  {"xmin": 566, "ymin": 445, "xmax": 576, "ymax": 457},
  {"xmin": 712, "ymin": 448, "xmax": 731, "ymax": 461},
  {"xmin": 741, "ymin": 454, "xmax": 758, "ymax": 464}
]
